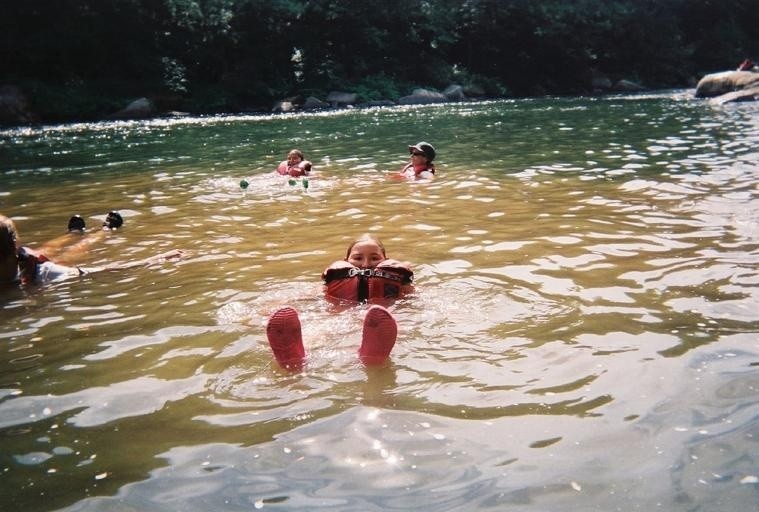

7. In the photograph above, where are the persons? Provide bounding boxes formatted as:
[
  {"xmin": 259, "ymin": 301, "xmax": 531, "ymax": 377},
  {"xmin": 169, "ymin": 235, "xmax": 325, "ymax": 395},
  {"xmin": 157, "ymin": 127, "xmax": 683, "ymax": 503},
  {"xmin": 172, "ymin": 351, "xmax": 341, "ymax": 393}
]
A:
[
  {"xmin": 266, "ymin": 232, "xmax": 415, "ymax": 372},
  {"xmin": 736, "ymin": 58, "xmax": 758, "ymax": 71},
  {"xmin": 276, "ymin": 149, "xmax": 314, "ymax": 176},
  {"xmin": 1, "ymin": 208, "xmax": 125, "ymax": 290},
  {"xmin": 393, "ymin": 141, "xmax": 436, "ymax": 180}
]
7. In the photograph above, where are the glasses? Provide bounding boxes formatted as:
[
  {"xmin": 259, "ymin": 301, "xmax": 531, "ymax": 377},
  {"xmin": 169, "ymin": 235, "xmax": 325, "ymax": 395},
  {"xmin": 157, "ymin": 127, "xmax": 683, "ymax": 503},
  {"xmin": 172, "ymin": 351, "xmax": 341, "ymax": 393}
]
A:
[{"xmin": 408, "ymin": 150, "xmax": 425, "ymax": 158}]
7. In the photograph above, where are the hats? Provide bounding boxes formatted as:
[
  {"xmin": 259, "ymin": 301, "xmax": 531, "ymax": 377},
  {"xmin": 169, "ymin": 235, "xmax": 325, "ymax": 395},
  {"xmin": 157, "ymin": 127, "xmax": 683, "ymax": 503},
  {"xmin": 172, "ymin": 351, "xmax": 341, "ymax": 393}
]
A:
[{"xmin": 408, "ymin": 142, "xmax": 436, "ymax": 162}]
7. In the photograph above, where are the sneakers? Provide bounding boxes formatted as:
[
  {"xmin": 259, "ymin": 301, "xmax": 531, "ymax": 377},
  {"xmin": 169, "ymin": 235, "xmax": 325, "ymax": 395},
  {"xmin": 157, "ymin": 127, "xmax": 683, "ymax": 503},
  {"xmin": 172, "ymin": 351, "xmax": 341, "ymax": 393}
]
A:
[
  {"xmin": 67, "ymin": 215, "xmax": 86, "ymax": 235},
  {"xmin": 358, "ymin": 305, "xmax": 397, "ymax": 367},
  {"xmin": 267, "ymin": 307, "xmax": 307, "ymax": 373},
  {"xmin": 102, "ymin": 211, "xmax": 123, "ymax": 228}
]
